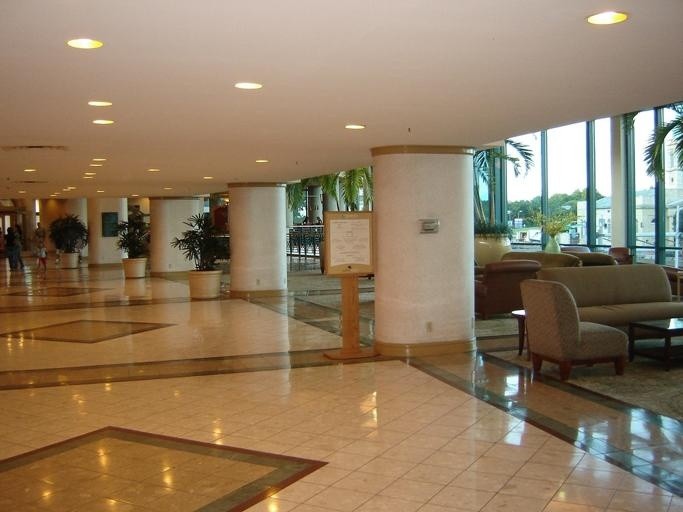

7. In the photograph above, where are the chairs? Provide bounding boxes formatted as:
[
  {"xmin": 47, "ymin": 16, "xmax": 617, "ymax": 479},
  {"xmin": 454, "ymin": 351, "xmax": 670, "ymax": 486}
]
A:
[
  {"xmin": 519, "ymin": 278, "xmax": 630, "ymax": 383},
  {"xmin": 475, "ymin": 259, "xmax": 543, "ymax": 320},
  {"xmin": 608, "ymin": 247, "xmax": 631, "ymax": 260},
  {"xmin": 561, "ymin": 246, "xmax": 590, "ymax": 252}
]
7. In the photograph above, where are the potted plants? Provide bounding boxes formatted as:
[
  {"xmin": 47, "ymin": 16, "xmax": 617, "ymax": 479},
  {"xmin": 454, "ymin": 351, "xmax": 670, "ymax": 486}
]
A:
[
  {"xmin": 168, "ymin": 212, "xmax": 230, "ymax": 300},
  {"xmin": 473, "ymin": 139, "xmax": 535, "ymax": 267},
  {"xmin": 111, "ymin": 204, "xmax": 152, "ymax": 278},
  {"xmin": 48, "ymin": 212, "xmax": 88, "ymax": 270}
]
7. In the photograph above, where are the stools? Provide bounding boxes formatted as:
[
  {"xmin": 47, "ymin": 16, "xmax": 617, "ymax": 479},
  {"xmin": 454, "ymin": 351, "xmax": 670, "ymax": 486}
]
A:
[{"xmin": 510, "ymin": 310, "xmax": 526, "ymax": 356}]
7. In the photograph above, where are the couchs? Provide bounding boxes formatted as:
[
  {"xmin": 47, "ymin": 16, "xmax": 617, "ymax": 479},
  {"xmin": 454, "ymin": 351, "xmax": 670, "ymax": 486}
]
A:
[{"xmin": 500, "ymin": 250, "xmax": 683, "ymax": 340}]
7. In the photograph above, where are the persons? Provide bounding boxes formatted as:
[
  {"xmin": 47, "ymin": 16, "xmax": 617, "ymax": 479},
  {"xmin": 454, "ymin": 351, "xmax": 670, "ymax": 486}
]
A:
[
  {"xmin": 303, "ymin": 217, "xmax": 311, "ymax": 225},
  {"xmin": 316, "ymin": 217, "xmax": 322, "ymax": 224},
  {"xmin": 4, "ymin": 223, "xmax": 47, "ymax": 272}
]
